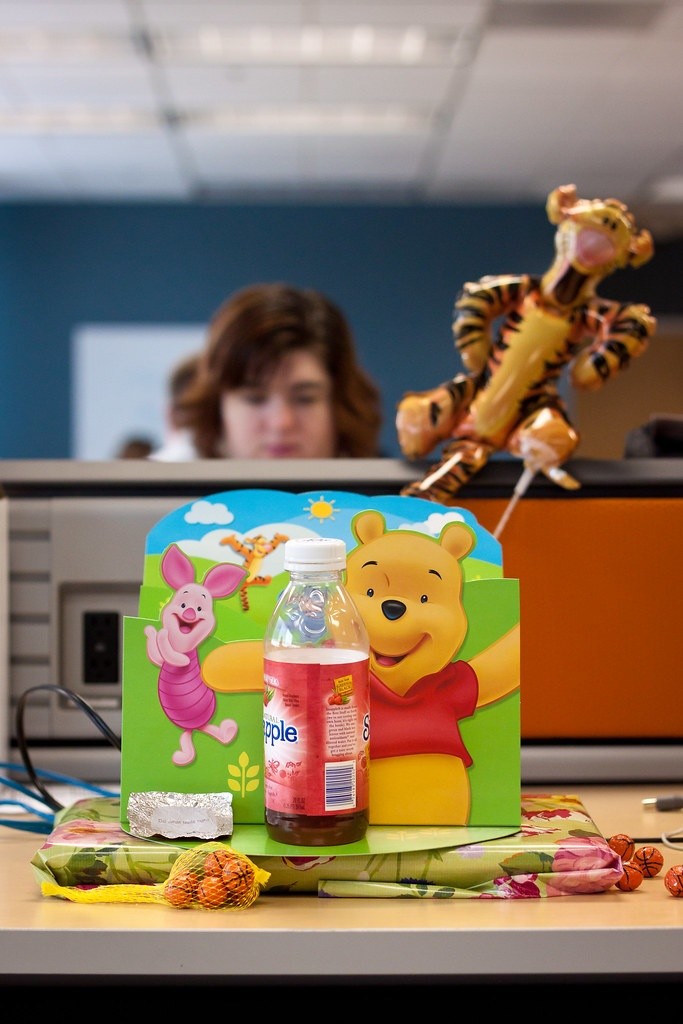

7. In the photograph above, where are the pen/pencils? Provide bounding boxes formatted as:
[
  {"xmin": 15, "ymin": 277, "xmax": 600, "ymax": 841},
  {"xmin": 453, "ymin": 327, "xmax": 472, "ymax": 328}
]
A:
[{"xmin": 641, "ymin": 795, "xmax": 682, "ymax": 811}]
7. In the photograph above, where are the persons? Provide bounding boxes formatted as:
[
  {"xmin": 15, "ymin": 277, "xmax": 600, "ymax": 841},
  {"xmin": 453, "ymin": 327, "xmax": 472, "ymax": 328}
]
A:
[{"xmin": 148, "ymin": 282, "xmax": 382, "ymax": 456}]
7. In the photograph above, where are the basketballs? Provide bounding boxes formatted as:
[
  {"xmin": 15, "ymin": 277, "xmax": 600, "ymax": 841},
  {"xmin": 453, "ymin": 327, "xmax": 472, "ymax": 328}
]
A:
[
  {"xmin": 664, "ymin": 865, "xmax": 683, "ymax": 898},
  {"xmin": 614, "ymin": 864, "xmax": 643, "ymax": 892},
  {"xmin": 162, "ymin": 849, "xmax": 260, "ymax": 910},
  {"xmin": 609, "ymin": 834, "xmax": 635, "ymax": 863},
  {"xmin": 633, "ymin": 846, "xmax": 663, "ymax": 877}
]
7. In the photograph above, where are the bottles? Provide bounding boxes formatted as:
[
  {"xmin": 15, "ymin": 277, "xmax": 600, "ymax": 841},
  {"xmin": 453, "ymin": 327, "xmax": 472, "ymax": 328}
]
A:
[{"xmin": 259, "ymin": 538, "xmax": 372, "ymax": 849}]
